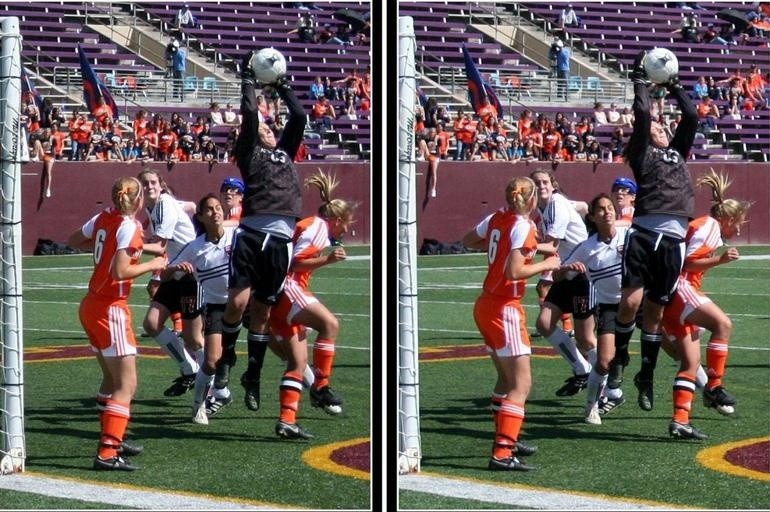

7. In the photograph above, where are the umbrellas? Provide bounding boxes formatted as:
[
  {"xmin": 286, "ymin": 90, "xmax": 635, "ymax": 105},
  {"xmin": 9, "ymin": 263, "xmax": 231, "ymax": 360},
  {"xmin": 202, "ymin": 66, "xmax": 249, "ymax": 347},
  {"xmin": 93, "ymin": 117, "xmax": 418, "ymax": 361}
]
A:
[
  {"xmin": 330, "ymin": 8, "xmax": 366, "ymax": 38},
  {"xmin": 711, "ymin": 9, "xmax": 756, "ymax": 40}
]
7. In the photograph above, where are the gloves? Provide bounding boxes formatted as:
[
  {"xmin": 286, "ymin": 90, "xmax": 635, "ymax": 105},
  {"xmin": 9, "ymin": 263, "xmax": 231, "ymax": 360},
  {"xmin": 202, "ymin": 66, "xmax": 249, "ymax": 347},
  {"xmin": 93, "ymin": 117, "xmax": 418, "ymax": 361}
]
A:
[
  {"xmin": 240, "ymin": 51, "xmax": 255, "ymax": 82},
  {"xmin": 631, "ymin": 50, "xmax": 647, "ymax": 81},
  {"xmin": 663, "ymin": 77, "xmax": 682, "ymax": 91},
  {"xmin": 273, "ymin": 76, "xmax": 290, "ymax": 91}
]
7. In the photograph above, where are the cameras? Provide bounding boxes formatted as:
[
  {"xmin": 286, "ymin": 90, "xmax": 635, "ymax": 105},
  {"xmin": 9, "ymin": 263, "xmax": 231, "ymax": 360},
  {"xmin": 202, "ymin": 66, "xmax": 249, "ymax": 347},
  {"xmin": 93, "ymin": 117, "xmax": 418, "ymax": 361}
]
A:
[
  {"xmin": 165, "ymin": 43, "xmax": 177, "ymax": 56},
  {"xmin": 550, "ymin": 43, "xmax": 562, "ymax": 53}
]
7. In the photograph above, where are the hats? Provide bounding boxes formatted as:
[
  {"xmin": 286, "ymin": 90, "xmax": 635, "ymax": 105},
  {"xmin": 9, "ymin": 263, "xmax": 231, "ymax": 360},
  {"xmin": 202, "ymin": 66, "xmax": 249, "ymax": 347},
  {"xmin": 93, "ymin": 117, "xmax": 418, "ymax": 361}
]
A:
[
  {"xmin": 221, "ymin": 177, "xmax": 246, "ymax": 192},
  {"xmin": 613, "ymin": 176, "xmax": 637, "ymax": 193}
]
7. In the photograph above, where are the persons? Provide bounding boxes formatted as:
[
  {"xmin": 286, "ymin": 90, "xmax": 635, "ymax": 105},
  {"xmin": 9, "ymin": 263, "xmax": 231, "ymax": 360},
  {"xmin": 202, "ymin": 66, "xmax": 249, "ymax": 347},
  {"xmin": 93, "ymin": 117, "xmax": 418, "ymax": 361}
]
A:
[
  {"xmin": 205, "ymin": 49, "xmax": 307, "ymax": 423},
  {"xmin": 170, "ymin": 40, "xmax": 186, "ymax": 101},
  {"xmin": 746, "ymin": 2, "xmax": 770, "ymax": 38},
  {"xmin": 171, "ymin": 82, "xmax": 205, "ymax": 164},
  {"xmin": 598, "ymin": 49, "xmax": 699, "ymax": 416},
  {"xmin": 349, "ymin": 19, "xmax": 370, "ymax": 45},
  {"xmin": 611, "ymin": 176, "xmax": 637, "ymax": 228},
  {"xmin": 559, "ymin": 2, "xmax": 579, "ymax": 32},
  {"xmin": 20, "ymin": 91, "xmax": 176, "ymax": 198},
  {"xmin": 116, "ymin": 79, "xmax": 132, "ymax": 95},
  {"xmin": 160, "ymin": 193, "xmax": 342, "ymax": 425},
  {"xmin": 415, "ymin": 95, "xmax": 557, "ymax": 198},
  {"xmin": 462, "ymin": 176, "xmax": 562, "ymax": 471},
  {"xmin": 554, "ymin": 38, "xmax": 569, "ymax": 100},
  {"xmin": 647, "ymin": 81, "xmax": 683, "ymax": 130},
  {"xmin": 531, "ymin": 216, "xmax": 574, "ymax": 336},
  {"xmin": 499, "ymin": 73, "xmax": 519, "ymax": 95},
  {"xmin": 719, "ymin": 23, "xmax": 770, "ymax": 48},
  {"xmin": 176, "ymin": 4, "xmax": 197, "ymax": 28},
  {"xmin": 286, "ymin": 2, "xmax": 344, "ymax": 48},
  {"xmin": 146, "ymin": 184, "xmax": 197, "ymax": 338},
  {"xmin": 256, "ymin": 84, "xmax": 281, "ymax": 134},
  {"xmin": 303, "ymin": 68, "xmax": 371, "ymax": 131},
  {"xmin": 659, "ymin": 168, "xmax": 746, "ymax": 441},
  {"xmin": 199, "ymin": 101, "xmax": 243, "ymax": 162},
  {"xmin": 67, "ymin": 176, "xmax": 169, "ymax": 471},
  {"xmin": 220, "ymin": 177, "xmax": 245, "ymax": 222},
  {"xmin": 552, "ymin": 193, "xmax": 736, "ymax": 426},
  {"xmin": 585, "ymin": 101, "xmax": 636, "ymax": 162},
  {"xmin": 528, "ymin": 168, "xmax": 627, "ymax": 415},
  {"xmin": 269, "ymin": 167, "xmax": 354, "ymax": 441},
  {"xmin": 689, "ymin": 62, "xmax": 769, "ymax": 128},
  {"xmin": 138, "ymin": 169, "xmax": 205, "ymax": 397},
  {"xmin": 546, "ymin": 100, "xmax": 590, "ymax": 171},
  {"xmin": 671, "ymin": 2, "xmax": 728, "ymax": 46}
]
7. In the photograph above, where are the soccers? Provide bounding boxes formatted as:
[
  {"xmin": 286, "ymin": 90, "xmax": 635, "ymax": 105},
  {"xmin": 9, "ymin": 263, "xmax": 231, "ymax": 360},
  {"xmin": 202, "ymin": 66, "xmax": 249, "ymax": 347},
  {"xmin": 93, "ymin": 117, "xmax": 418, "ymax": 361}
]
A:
[
  {"xmin": 252, "ymin": 47, "xmax": 287, "ymax": 83},
  {"xmin": 644, "ymin": 47, "xmax": 679, "ymax": 83}
]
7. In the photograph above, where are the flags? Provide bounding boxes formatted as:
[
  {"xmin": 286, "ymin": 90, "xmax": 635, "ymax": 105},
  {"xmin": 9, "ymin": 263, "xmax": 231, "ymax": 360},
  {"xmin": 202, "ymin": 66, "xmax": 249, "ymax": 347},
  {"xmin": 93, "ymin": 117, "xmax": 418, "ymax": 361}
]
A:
[
  {"xmin": 78, "ymin": 39, "xmax": 119, "ymax": 122},
  {"xmin": 416, "ymin": 85, "xmax": 439, "ymax": 120},
  {"xmin": 460, "ymin": 41, "xmax": 505, "ymax": 122}
]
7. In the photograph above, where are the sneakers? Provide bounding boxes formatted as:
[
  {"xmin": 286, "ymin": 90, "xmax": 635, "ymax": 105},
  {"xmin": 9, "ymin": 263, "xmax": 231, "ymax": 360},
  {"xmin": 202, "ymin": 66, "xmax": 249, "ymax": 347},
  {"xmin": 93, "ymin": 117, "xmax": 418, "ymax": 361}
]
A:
[
  {"xmin": 191, "ymin": 400, "xmax": 210, "ymax": 426},
  {"xmin": 702, "ymin": 384, "xmax": 737, "ymax": 407},
  {"xmin": 431, "ymin": 190, "xmax": 437, "ymax": 197},
  {"xmin": 633, "ymin": 373, "xmax": 655, "ymax": 412},
  {"xmin": 669, "ymin": 419, "xmax": 709, "ymax": 441},
  {"xmin": 45, "ymin": 189, "xmax": 50, "ymax": 198},
  {"xmin": 417, "ymin": 156, "xmax": 425, "ymax": 161},
  {"xmin": 274, "ymin": 420, "xmax": 315, "ymax": 441},
  {"xmin": 239, "ymin": 372, "xmax": 262, "ymax": 411},
  {"xmin": 163, "ymin": 372, "xmax": 196, "ymax": 397},
  {"xmin": 585, "ymin": 400, "xmax": 602, "ymax": 426},
  {"xmin": 309, "ymin": 384, "xmax": 344, "ymax": 408},
  {"xmin": 93, "ymin": 455, "xmax": 142, "ymax": 472},
  {"xmin": 555, "ymin": 373, "xmax": 590, "ymax": 397},
  {"xmin": 488, "ymin": 456, "xmax": 537, "ymax": 471},
  {"xmin": 117, "ymin": 442, "xmax": 144, "ymax": 458},
  {"xmin": 213, "ymin": 353, "xmax": 237, "ymax": 390},
  {"xmin": 514, "ymin": 440, "xmax": 538, "ymax": 456},
  {"xmin": 596, "ymin": 393, "xmax": 626, "ymax": 417},
  {"xmin": 204, "ymin": 390, "xmax": 233, "ymax": 417},
  {"xmin": 31, "ymin": 155, "xmax": 40, "ymax": 162},
  {"xmin": 606, "ymin": 354, "xmax": 630, "ymax": 390}
]
